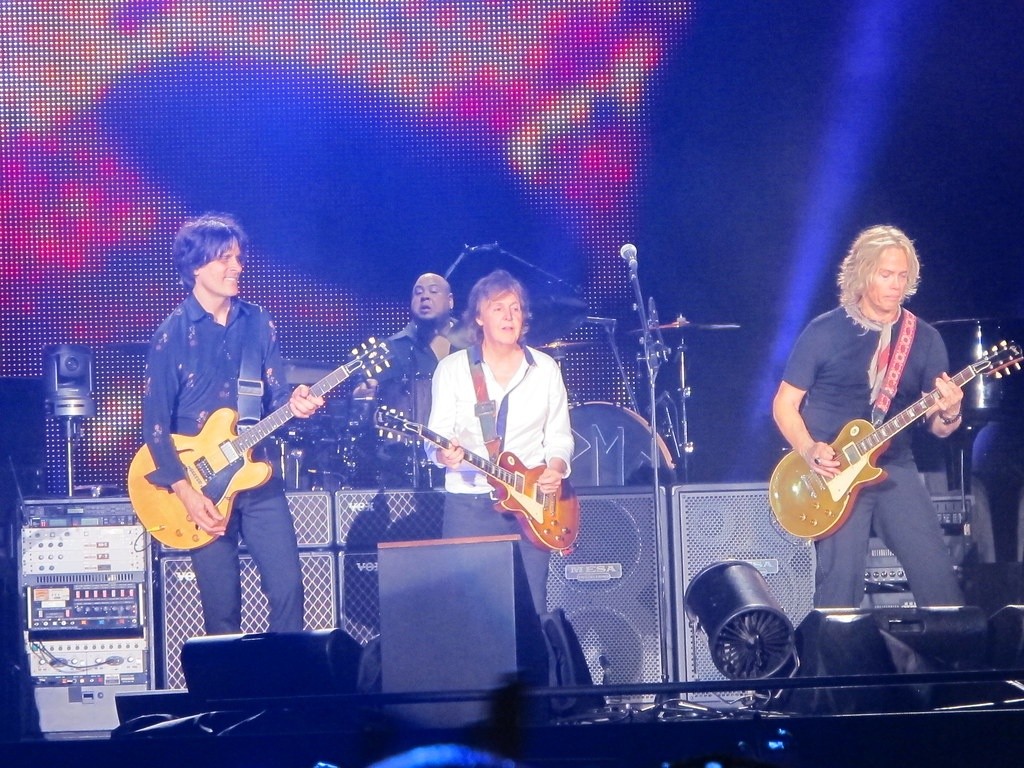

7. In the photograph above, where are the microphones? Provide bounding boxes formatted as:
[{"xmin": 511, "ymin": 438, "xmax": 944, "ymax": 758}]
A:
[{"xmin": 620, "ymin": 243, "xmax": 638, "ymax": 268}]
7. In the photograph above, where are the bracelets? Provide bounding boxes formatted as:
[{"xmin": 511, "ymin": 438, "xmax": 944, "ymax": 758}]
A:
[{"xmin": 939, "ymin": 403, "xmax": 962, "ymax": 424}]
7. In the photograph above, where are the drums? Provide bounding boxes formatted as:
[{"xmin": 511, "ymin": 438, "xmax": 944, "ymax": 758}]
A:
[
  {"xmin": 568, "ymin": 401, "xmax": 677, "ymax": 488},
  {"xmin": 376, "ymin": 371, "xmax": 431, "ymax": 449}
]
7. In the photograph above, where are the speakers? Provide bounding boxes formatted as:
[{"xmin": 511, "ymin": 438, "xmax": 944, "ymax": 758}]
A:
[
  {"xmin": 181, "ymin": 628, "xmax": 361, "ymax": 694},
  {"xmin": 335, "ymin": 490, "xmax": 447, "ymax": 548},
  {"xmin": 160, "ymin": 551, "xmax": 337, "ymax": 691},
  {"xmin": 988, "ymin": 604, "xmax": 1024, "ymax": 671},
  {"xmin": 753, "ymin": 605, "xmax": 989, "ymax": 715},
  {"xmin": 671, "ymin": 484, "xmax": 819, "ymax": 703},
  {"xmin": 338, "ymin": 551, "xmax": 380, "ymax": 645},
  {"xmin": 158, "ymin": 490, "xmax": 333, "ymax": 552},
  {"xmin": 546, "ymin": 486, "xmax": 673, "ymax": 704}
]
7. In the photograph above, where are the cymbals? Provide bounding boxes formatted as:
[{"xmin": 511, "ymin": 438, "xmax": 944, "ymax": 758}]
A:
[
  {"xmin": 626, "ymin": 322, "xmax": 741, "ymax": 334},
  {"xmin": 525, "ymin": 295, "xmax": 592, "ymax": 344},
  {"xmin": 532, "ymin": 340, "xmax": 594, "ymax": 356}
]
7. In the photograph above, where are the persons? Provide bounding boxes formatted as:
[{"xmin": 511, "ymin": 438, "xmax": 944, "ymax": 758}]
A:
[
  {"xmin": 424, "ymin": 269, "xmax": 575, "ymax": 616},
  {"xmin": 141, "ymin": 216, "xmax": 323, "ymax": 637},
  {"xmin": 772, "ymin": 227, "xmax": 964, "ymax": 610},
  {"xmin": 355, "ymin": 272, "xmax": 478, "ymax": 425}
]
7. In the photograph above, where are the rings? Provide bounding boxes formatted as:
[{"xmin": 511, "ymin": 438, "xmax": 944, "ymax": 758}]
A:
[{"xmin": 815, "ymin": 457, "xmax": 821, "ymax": 466}]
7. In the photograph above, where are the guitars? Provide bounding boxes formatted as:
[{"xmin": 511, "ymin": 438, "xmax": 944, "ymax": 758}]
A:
[
  {"xmin": 769, "ymin": 340, "xmax": 1024, "ymax": 542},
  {"xmin": 128, "ymin": 336, "xmax": 391, "ymax": 550},
  {"xmin": 373, "ymin": 405, "xmax": 581, "ymax": 555}
]
